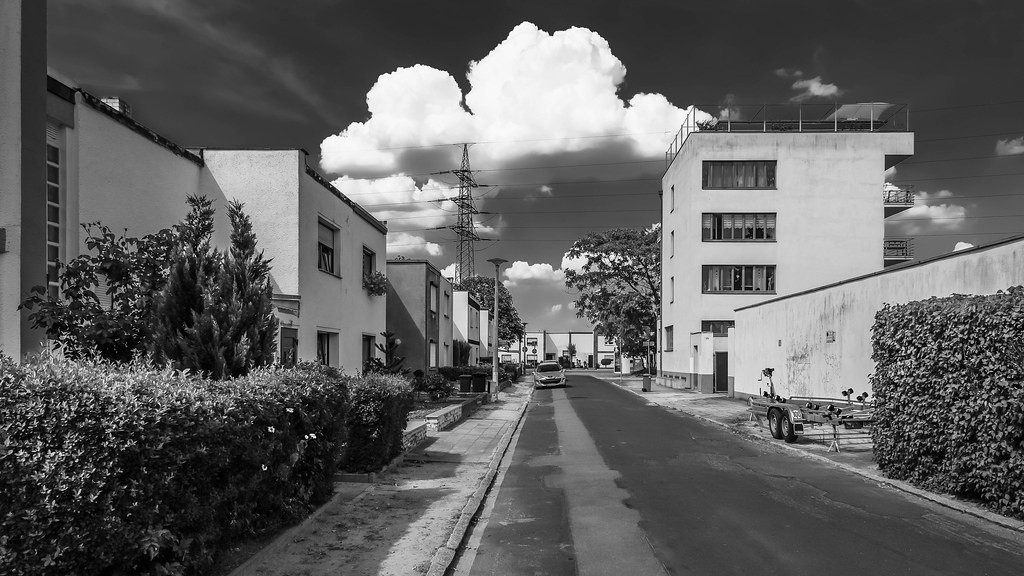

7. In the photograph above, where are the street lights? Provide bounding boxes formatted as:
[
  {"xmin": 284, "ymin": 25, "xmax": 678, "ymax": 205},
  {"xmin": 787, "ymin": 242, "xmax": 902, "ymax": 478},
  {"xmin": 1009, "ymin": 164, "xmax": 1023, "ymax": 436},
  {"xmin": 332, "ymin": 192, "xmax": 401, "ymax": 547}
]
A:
[
  {"xmin": 486, "ymin": 258, "xmax": 508, "ymax": 402},
  {"xmin": 521, "ymin": 321, "xmax": 529, "ymax": 375}
]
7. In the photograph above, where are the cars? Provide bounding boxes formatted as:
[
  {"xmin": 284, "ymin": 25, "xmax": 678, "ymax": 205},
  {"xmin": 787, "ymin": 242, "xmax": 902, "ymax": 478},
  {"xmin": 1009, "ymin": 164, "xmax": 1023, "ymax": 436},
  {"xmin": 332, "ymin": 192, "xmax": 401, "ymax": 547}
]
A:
[
  {"xmin": 544, "ymin": 360, "xmax": 563, "ymax": 372},
  {"xmin": 532, "ymin": 363, "xmax": 566, "ymax": 390}
]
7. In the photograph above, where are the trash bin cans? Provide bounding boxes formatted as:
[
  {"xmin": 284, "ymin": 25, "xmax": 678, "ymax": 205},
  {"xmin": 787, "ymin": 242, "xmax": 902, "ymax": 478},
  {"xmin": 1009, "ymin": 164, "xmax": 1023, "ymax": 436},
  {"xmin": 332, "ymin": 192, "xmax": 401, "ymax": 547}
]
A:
[
  {"xmin": 472, "ymin": 373, "xmax": 488, "ymax": 393},
  {"xmin": 642, "ymin": 374, "xmax": 652, "ymax": 392},
  {"xmin": 458, "ymin": 374, "xmax": 472, "ymax": 392}
]
